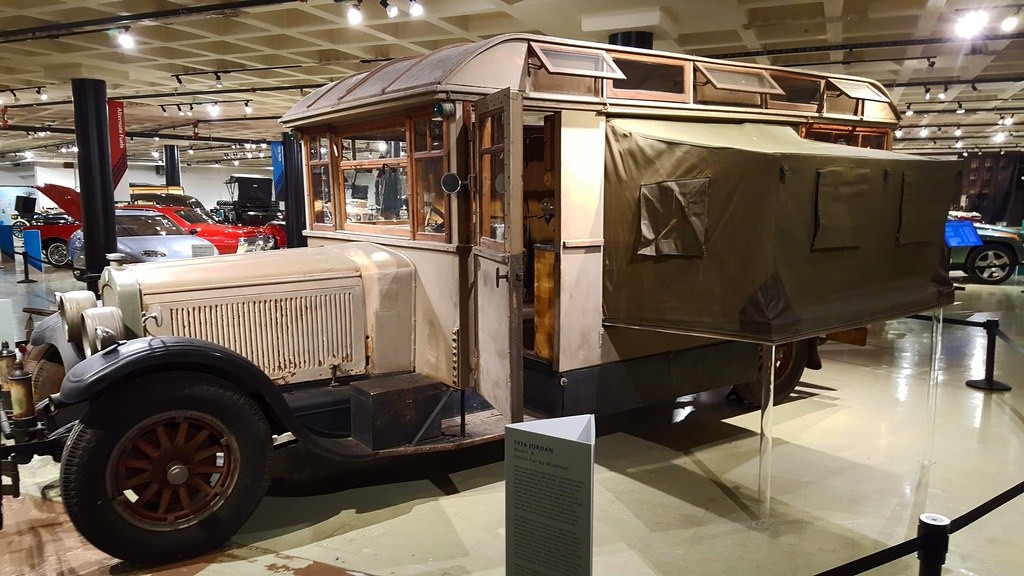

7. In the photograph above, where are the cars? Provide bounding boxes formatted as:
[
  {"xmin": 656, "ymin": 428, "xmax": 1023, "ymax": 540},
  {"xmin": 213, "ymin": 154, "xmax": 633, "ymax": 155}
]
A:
[
  {"xmin": 66, "ymin": 212, "xmax": 219, "ymax": 281},
  {"xmin": 22, "ymin": 183, "xmax": 281, "ymax": 268},
  {"xmin": 344, "ymin": 198, "xmax": 376, "ymax": 222},
  {"xmin": 947, "ymin": 214, "xmax": 1024, "ymax": 285},
  {"xmin": 379, "ymin": 195, "xmax": 410, "ymax": 221}
]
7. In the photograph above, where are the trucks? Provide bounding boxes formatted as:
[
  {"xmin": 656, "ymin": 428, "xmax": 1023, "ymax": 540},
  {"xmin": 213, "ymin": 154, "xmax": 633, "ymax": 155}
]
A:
[
  {"xmin": 0, "ymin": 33, "xmax": 967, "ymax": 565},
  {"xmin": 43, "ymin": 193, "xmax": 231, "ymax": 226}
]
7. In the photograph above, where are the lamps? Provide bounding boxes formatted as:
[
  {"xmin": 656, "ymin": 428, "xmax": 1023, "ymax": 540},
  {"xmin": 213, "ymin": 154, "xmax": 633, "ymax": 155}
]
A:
[
  {"xmin": 214, "ymin": 73, "xmax": 224, "ymax": 88},
  {"xmin": 244, "ymin": 101, "xmax": 251, "ymax": 113},
  {"xmin": 36, "ymin": 88, "xmax": 48, "ymax": 101},
  {"xmin": 175, "ymin": 75, "xmax": 190, "ymax": 93},
  {"xmin": 10, "ymin": 91, "xmax": 21, "ymax": 103},
  {"xmin": 379, "ymin": 0, "xmax": 398, "ymax": 17},
  {"xmin": 176, "ymin": 105, "xmax": 184, "ymax": 116},
  {"xmin": 409, "ymin": 0, "xmax": 423, "ymax": 17},
  {"xmin": 161, "ymin": 106, "xmax": 170, "ymax": 116},
  {"xmin": 188, "ymin": 146, "xmax": 194, "ymax": 154},
  {"xmin": 348, "ymin": 0, "xmax": 363, "ymax": 26},
  {"xmin": 186, "ymin": 104, "xmax": 194, "ymax": 116}
]
